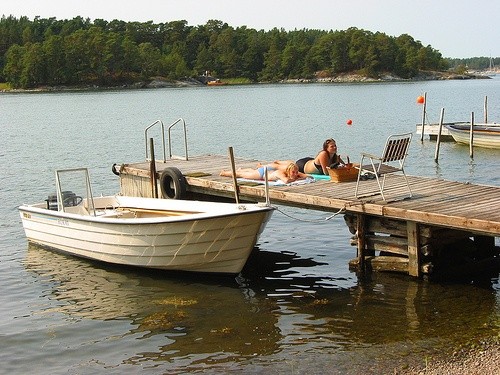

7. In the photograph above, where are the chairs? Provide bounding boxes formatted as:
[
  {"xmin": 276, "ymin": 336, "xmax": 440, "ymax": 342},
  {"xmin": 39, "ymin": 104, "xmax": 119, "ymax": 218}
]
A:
[{"xmin": 354, "ymin": 132, "xmax": 412, "ymax": 204}]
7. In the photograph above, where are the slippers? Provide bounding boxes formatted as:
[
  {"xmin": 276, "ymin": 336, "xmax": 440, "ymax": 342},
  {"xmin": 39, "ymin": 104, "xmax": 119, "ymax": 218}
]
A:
[
  {"xmin": 360, "ymin": 171, "xmax": 370, "ymax": 181},
  {"xmin": 368, "ymin": 171, "xmax": 380, "ymax": 179}
]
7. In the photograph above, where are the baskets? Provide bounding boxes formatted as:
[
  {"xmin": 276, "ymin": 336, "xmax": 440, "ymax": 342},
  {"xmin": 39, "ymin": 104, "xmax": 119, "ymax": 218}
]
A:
[{"xmin": 327, "ymin": 163, "xmax": 360, "ymax": 183}]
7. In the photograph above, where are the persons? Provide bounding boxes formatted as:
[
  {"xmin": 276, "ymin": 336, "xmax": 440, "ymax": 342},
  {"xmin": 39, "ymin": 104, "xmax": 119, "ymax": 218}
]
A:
[
  {"xmin": 255, "ymin": 137, "xmax": 346, "ymax": 176},
  {"xmin": 219, "ymin": 161, "xmax": 314, "ymax": 184}
]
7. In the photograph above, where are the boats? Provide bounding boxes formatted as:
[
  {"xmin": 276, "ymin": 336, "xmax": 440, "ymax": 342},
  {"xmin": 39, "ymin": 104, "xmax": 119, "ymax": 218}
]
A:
[
  {"xmin": 445, "ymin": 124, "xmax": 500, "ymax": 150},
  {"xmin": 18, "ymin": 166, "xmax": 278, "ymax": 275},
  {"xmin": 207, "ymin": 79, "xmax": 228, "ymax": 86}
]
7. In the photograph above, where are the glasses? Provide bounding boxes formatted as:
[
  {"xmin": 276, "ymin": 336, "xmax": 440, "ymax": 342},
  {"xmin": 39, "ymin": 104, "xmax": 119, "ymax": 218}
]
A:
[{"xmin": 326, "ymin": 139, "xmax": 336, "ymax": 144}]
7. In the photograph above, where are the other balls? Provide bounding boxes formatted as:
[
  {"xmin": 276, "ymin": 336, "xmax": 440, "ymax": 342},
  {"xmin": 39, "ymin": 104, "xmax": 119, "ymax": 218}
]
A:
[
  {"xmin": 417, "ymin": 96, "xmax": 424, "ymax": 103},
  {"xmin": 347, "ymin": 120, "xmax": 352, "ymax": 124}
]
